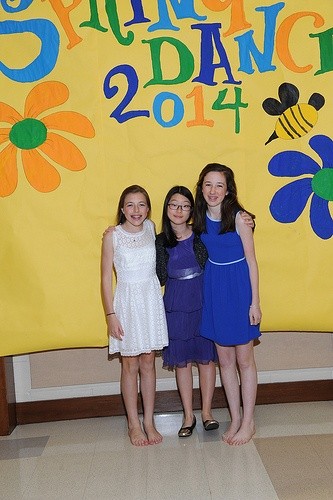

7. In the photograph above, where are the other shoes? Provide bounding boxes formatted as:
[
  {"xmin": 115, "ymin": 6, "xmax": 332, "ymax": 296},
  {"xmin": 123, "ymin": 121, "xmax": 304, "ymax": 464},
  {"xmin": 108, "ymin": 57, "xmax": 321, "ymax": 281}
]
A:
[
  {"xmin": 202, "ymin": 419, "xmax": 219, "ymax": 430},
  {"xmin": 178, "ymin": 414, "xmax": 196, "ymax": 437}
]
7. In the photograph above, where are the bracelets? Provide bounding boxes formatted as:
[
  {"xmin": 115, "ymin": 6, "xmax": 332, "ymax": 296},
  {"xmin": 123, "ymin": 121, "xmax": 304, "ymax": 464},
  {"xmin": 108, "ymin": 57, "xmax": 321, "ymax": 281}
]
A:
[
  {"xmin": 249, "ymin": 304, "xmax": 260, "ymax": 309},
  {"xmin": 105, "ymin": 312, "xmax": 116, "ymax": 316}
]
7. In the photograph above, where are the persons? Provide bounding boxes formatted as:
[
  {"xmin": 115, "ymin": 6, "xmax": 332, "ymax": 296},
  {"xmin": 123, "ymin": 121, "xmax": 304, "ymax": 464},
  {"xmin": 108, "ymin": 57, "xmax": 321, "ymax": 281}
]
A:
[
  {"xmin": 193, "ymin": 163, "xmax": 263, "ymax": 446},
  {"xmin": 102, "ymin": 186, "xmax": 255, "ymax": 437},
  {"xmin": 99, "ymin": 184, "xmax": 169, "ymax": 446}
]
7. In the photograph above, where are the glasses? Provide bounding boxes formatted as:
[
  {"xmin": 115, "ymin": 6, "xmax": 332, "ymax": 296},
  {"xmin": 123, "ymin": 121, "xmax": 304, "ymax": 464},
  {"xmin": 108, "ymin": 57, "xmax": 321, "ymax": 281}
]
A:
[{"xmin": 167, "ymin": 203, "xmax": 193, "ymax": 211}]
